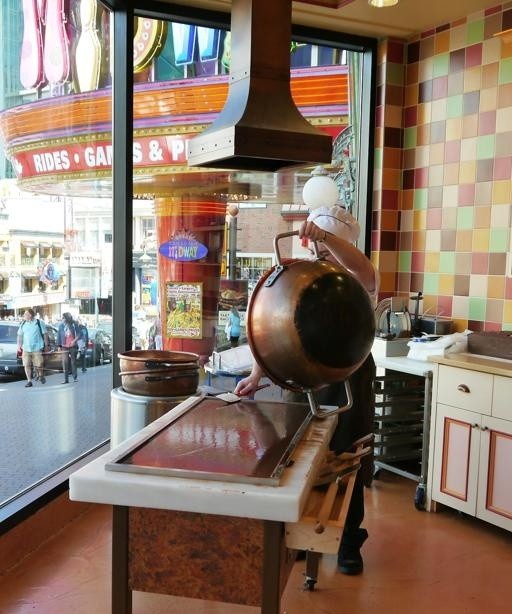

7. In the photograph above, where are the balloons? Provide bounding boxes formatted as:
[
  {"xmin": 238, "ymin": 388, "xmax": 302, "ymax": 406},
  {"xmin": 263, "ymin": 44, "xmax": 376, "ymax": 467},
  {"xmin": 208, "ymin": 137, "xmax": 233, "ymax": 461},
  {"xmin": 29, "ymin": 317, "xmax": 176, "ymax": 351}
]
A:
[{"xmin": 35, "ymin": 259, "xmax": 61, "ymax": 290}]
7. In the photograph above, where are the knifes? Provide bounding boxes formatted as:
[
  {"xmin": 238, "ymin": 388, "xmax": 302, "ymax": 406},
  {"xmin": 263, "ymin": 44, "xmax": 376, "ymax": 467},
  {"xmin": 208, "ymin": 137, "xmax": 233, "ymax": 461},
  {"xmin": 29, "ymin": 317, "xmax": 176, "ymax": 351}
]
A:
[{"xmin": 312, "ymin": 433, "xmax": 375, "ymax": 490}]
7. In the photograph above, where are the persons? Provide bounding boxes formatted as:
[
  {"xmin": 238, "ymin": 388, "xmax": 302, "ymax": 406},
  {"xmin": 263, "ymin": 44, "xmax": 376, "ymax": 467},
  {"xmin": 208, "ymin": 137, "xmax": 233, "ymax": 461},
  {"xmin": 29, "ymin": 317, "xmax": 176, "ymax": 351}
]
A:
[
  {"xmin": 16, "ymin": 308, "xmax": 51, "ymax": 387},
  {"xmin": 133, "ymin": 333, "xmax": 142, "ymax": 350},
  {"xmin": 228, "ymin": 202, "xmax": 383, "ymax": 576},
  {"xmin": 230, "ymin": 398, "xmax": 310, "ymax": 456},
  {"xmin": 32, "ymin": 284, "xmax": 44, "ymax": 293},
  {"xmin": 79, "ymin": 322, "xmax": 87, "ymax": 372},
  {"xmin": 226, "ymin": 307, "xmax": 242, "ymax": 350},
  {"xmin": 56, "ymin": 311, "xmax": 83, "ymax": 384}
]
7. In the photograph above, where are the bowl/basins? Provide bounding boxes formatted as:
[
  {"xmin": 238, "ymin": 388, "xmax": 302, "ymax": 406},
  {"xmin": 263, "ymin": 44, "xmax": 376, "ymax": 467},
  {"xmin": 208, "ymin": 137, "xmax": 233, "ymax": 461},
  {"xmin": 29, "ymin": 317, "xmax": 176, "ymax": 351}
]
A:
[{"xmin": 377, "ymin": 298, "xmax": 411, "ymax": 340}]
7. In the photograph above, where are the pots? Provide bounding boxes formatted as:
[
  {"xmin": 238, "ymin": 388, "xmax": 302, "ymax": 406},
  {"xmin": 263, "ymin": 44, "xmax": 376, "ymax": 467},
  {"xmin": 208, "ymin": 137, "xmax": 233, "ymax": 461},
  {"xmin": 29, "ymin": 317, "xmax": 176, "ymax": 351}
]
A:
[
  {"xmin": 246, "ymin": 230, "xmax": 377, "ymax": 419},
  {"xmin": 116, "ymin": 349, "xmax": 199, "ymax": 397}
]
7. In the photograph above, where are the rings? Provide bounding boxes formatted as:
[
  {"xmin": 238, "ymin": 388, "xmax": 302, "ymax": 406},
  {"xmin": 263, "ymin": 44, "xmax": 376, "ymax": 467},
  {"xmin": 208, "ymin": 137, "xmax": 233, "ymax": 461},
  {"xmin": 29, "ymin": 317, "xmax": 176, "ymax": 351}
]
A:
[{"xmin": 312, "ymin": 224, "xmax": 318, "ymax": 228}]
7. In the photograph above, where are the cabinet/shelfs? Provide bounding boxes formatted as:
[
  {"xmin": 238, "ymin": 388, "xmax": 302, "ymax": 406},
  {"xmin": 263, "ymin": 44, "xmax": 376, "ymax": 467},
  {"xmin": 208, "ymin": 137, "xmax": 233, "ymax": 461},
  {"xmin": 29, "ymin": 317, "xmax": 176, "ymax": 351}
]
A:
[{"xmin": 434, "ymin": 362, "xmax": 511, "ymax": 532}]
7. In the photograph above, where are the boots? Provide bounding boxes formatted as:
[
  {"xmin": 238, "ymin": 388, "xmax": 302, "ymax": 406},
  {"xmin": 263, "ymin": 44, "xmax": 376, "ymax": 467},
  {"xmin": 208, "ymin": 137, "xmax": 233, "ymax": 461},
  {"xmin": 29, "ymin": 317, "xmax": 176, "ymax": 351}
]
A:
[{"xmin": 335, "ymin": 526, "xmax": 369, "ymax": 575}]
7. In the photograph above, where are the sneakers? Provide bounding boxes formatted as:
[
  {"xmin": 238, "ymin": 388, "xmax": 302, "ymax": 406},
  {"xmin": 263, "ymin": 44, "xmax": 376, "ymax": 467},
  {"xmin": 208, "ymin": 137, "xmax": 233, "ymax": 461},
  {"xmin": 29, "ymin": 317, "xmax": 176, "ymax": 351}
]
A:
[
  {"xmin": 60, "ymin": 378, "xmax": 78, "ymax": 384},
  {"xmin": 23, "ymin": 375, "xmax": 46, "ymax": 387}
]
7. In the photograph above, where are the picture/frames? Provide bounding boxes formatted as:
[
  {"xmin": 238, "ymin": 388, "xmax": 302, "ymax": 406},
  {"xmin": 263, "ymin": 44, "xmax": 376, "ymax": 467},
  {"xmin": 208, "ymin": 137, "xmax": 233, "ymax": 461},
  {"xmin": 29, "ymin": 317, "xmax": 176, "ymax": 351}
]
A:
[{"xmin": 162, "ymin": 280, "xmax": 203, "ymax": 340}]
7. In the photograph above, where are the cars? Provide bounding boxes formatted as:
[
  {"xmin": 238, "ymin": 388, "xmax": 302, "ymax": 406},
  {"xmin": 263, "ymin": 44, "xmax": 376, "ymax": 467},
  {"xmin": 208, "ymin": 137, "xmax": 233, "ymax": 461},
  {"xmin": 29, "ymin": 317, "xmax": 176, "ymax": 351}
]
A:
[
  {"xmin": 0, "ymin": 319, "xmax": 81, "ymax": 381},
  {"xmin": 85, "ymin": 327, "xmax": 112, "ymax": 367}
]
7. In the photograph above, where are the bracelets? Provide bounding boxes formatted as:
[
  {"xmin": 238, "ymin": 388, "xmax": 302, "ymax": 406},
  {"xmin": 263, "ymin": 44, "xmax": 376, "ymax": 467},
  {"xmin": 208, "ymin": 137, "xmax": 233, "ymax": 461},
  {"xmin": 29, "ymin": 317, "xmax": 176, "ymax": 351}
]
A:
[
  {"xmin": 318, "ymin": 229, "xmax": 327, "ymax": 245},
  {"xmin": 44, "ymin": 345, "xmax": 49, "ymax": 347}
]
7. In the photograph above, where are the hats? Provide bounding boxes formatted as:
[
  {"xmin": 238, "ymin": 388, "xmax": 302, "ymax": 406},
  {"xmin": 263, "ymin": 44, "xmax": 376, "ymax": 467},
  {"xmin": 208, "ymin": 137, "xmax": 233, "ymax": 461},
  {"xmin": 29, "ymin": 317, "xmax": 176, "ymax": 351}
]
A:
[{"xmin": 304, "ymin": 205, "xmax": 361, "ymax": 246}]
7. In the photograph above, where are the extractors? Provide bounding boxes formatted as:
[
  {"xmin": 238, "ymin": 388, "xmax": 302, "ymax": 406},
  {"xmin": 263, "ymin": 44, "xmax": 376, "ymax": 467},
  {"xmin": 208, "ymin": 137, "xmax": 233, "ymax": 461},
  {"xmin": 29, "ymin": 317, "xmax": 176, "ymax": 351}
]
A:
[{"xmin": 184, "ymin": 0, "xmax": 333, "ymax": 177}]
